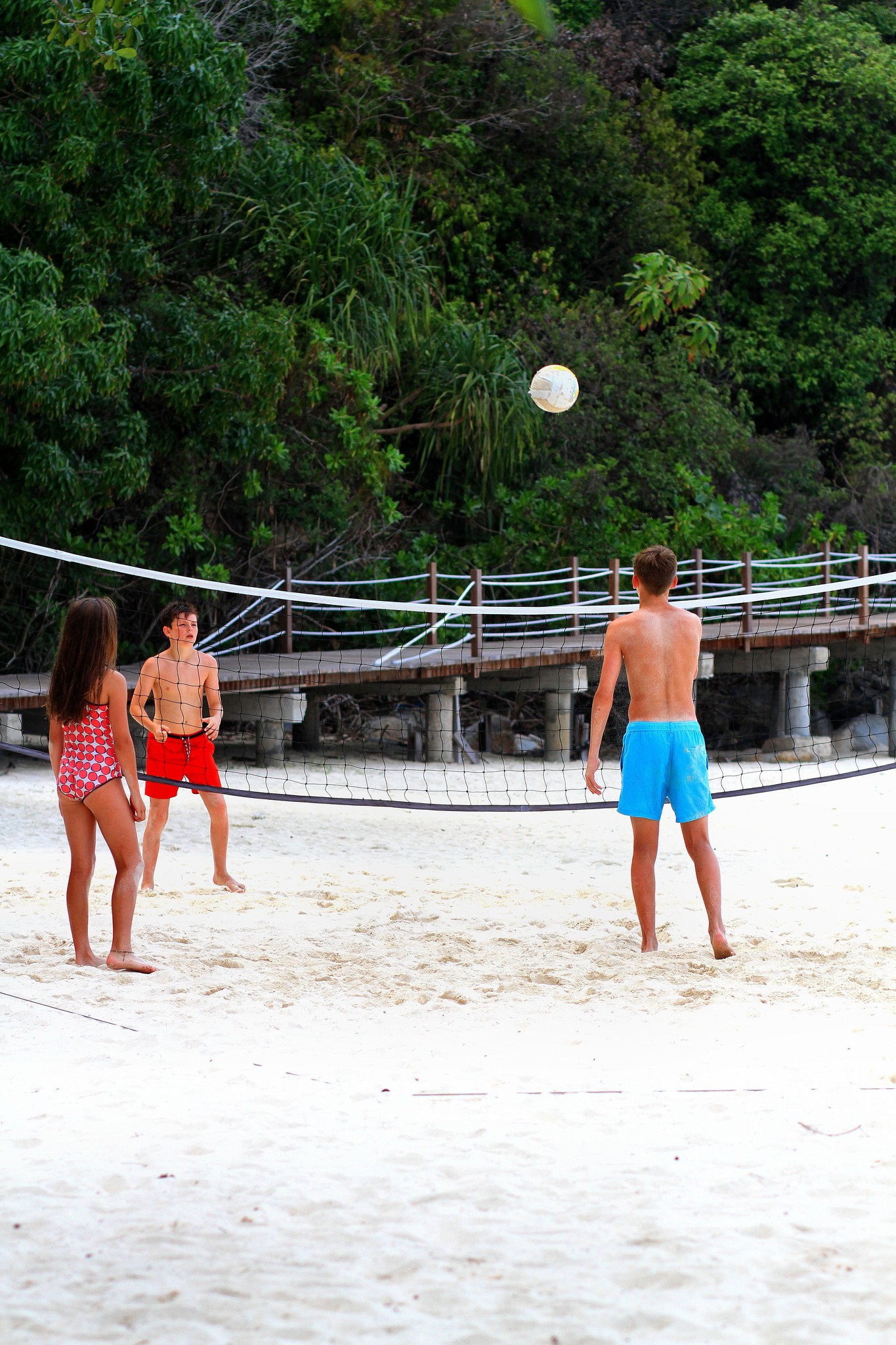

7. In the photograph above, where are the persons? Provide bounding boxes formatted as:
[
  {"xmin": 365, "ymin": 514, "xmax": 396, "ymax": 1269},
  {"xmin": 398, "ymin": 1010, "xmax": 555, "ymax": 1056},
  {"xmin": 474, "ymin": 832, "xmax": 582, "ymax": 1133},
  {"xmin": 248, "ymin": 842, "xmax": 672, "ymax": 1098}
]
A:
[
  {"xmin": 583, "ymin": 545, "xmax": 735, "ymax": 960},
  {"xmin": 44, "ymin": 595, "xmax": 161, "ymax": 974},
  {"xmin": 128, "ymin": 600, "xmax": 247, "ymax": 894}
]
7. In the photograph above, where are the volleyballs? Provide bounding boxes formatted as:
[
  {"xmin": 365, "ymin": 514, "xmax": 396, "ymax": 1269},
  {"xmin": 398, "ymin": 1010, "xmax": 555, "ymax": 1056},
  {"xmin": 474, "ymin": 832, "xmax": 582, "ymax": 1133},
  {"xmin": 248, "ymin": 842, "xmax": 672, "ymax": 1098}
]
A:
[{"xmin": 527, "ymin": 364, "xmax": 579, "ymax": 414}]
